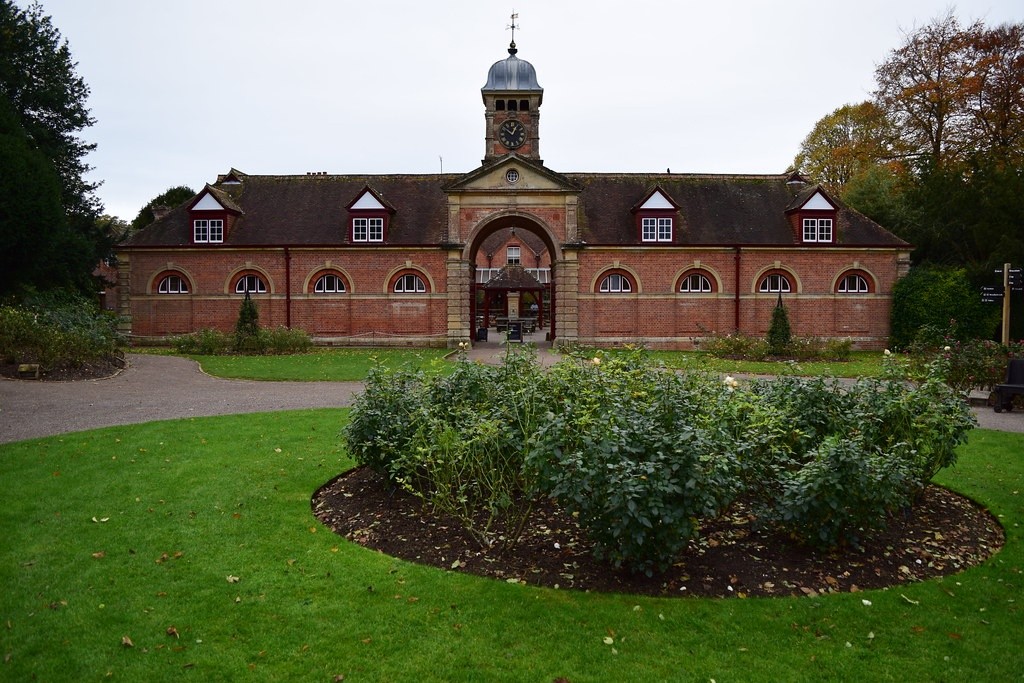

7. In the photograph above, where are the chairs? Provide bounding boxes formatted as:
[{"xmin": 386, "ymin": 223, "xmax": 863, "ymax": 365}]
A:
[
  {"xmin": 507, "ymin": 322, "xmax": 524, "ymax": 343},
  {"xmin": 524, "ymin": 320, "xmax": 534, "ymax": 335}
]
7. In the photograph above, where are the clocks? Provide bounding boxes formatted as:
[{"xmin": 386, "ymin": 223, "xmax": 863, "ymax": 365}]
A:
[{"xmin": 498, "ymin": 119, "xmax": 527, "ymax": 150}]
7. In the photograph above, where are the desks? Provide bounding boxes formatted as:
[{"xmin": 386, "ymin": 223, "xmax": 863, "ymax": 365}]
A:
[{"xmin": 495, "ymin": 318, "xmax": 538, "ymax": 334}]
